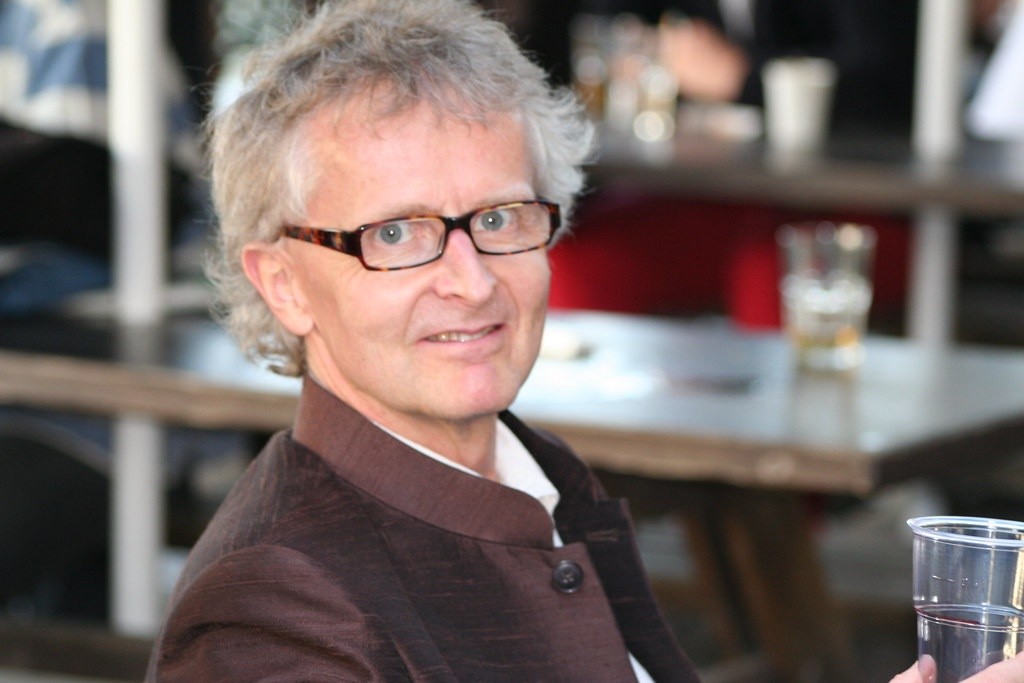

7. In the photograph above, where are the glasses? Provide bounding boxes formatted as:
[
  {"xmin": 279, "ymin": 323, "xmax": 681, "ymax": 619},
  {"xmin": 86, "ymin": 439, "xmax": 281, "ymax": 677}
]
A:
[{"xmin": 281, "ymin": 201, "xmax": 562, "ymax": 270}]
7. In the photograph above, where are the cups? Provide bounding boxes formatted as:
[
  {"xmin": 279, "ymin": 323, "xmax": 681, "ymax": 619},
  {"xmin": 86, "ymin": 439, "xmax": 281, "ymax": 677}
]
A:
[
  {"xmin": 778, "ymin": 223, "xmax": 875, "ymax": 369},
  {"xmin": 907, "ymin": 514, "xmax": 1024, "ymax": 683},
  {"xmin": 764, "ymin": 59, "xmax": 836, "ymax": 163}
]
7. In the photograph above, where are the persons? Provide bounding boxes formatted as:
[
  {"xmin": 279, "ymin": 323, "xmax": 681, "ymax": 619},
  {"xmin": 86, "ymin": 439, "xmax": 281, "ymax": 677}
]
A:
[
  {"xmin": 515, "ymin": 0, "xmax": 1023, "ymax": 349},
  {"xmin": 140, "ymin": 0, "xmax": 1023, "ymax": 682},
  {"xmin": 0, "ymin": 0, "xmax": 204, "ymax": 624}
]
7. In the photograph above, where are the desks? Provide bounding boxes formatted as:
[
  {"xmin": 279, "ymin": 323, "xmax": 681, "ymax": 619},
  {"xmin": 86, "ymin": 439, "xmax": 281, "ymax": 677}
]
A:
[{"xmin": 0, "ymin": 312, "xmax": 1024, "ymax": 502}]
